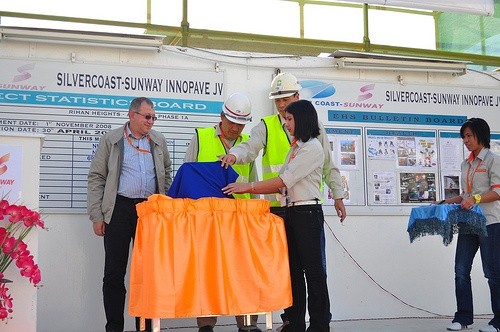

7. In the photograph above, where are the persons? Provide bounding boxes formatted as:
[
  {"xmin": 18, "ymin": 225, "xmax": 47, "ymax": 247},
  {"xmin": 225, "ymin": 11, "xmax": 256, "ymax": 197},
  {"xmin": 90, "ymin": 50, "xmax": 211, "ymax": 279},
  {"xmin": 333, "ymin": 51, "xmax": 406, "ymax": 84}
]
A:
[
  {"xmin": 87, "ymin": 97, "xmax": 173, "ymax": 332},
  {"xmin": 221, "ymin": 100, "xmax": 332, "ymax": 332},
  {"xmin": 184, "ymin": 94, "xmax": 264, "ymax": 332},
  {"xmin": 217, "ymin": 72, "xmax": 346, "ymax": 332},
  {"xmin": 431, "ymin": 117, "xmax": 500, "ymax": 332}
]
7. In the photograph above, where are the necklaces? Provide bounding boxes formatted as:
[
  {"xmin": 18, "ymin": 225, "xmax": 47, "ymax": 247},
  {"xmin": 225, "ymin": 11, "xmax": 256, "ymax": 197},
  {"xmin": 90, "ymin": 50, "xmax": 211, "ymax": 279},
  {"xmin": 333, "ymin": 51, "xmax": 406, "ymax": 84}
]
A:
[
  {"xmin": 466, "ymin": 159, "xmax": 482, "ymax": 198},
  {"xmin": 124, "ymin": 126, "xmax": 152, "ymax": 153},
  {"xmin": 219, "ymin": 122, "xmax": 241, "ymax": 150}
]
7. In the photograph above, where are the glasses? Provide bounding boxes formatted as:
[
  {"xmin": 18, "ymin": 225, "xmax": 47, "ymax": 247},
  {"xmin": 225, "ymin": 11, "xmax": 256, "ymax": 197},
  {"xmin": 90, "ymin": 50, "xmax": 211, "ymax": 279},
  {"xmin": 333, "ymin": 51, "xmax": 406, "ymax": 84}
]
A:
[{"xmin": 134, "ymin": 111, "xmax": 157, "ymax": 121}]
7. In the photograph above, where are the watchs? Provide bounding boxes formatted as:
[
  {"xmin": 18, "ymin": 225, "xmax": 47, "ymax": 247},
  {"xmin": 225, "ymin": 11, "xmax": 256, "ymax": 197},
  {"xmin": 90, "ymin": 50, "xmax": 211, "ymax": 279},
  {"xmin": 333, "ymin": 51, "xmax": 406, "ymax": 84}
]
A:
[{"xmin": 472, "ymin": 194, "xmax": 481, "ymax": 203}]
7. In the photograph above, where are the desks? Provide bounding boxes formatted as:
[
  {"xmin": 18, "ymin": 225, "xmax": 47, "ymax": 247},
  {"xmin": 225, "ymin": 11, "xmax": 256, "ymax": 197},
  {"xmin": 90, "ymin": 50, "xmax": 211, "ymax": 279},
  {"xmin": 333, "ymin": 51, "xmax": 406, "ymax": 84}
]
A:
[{"xmin": 127, "ymin": 194, "xmax": 293, "ymax": 332}]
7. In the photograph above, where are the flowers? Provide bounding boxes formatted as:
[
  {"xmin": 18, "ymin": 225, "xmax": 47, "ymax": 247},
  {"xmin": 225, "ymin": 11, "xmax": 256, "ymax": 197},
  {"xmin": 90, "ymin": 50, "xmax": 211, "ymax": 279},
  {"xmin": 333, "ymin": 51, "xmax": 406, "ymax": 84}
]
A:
[{"xmin": 0, "ymin": 199, "xmax": 50, "ymax": 325}]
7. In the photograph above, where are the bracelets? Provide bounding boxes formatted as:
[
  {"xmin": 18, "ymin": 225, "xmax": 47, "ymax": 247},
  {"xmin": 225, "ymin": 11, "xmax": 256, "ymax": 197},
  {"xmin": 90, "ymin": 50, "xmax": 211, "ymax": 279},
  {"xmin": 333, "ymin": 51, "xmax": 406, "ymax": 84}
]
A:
[{"xmin": 251, "ymin": 182, "xmax": 255, "ymax": 194}]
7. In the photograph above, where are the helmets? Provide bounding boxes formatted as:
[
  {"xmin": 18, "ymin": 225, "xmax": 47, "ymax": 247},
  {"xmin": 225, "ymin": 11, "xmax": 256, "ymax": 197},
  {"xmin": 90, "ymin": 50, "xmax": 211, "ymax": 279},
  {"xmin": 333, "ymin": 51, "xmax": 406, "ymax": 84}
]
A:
[
  {"xmin": 268, "ymin": 73, "xmax": 302, "ymax": 99},
  {"xmin": 222, "ymin": 93, "xmax": 252, "ymax": 124}
]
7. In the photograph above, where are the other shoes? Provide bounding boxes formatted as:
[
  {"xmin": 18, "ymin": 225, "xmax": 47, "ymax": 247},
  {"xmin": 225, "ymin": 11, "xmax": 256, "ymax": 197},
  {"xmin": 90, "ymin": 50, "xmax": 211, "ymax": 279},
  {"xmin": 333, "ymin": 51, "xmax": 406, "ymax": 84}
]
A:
[
  {"xmin": 238, "ymin": 328, "xmax": 262, "ymax": 332},
  {"xmin": 281, "ymin": 323, "xmax": 306, "ymax": 332},
  {"xmin": 446, "ymin": 321, "xmax": 474, "ymax": 330},
  {"xmin": 305, "ymin": 325, "xmax": 330, "ymax": 332},
  {"xmin": 479, "ymin": 324, "xmax": 500, "ymax": 332},
  {"xmin": 275, "ymin": 321, "xmax": 289, "ymax": 332},
  {"xmin": 198, "ymin": 326, "xmax": 214, "ymax": 332}
]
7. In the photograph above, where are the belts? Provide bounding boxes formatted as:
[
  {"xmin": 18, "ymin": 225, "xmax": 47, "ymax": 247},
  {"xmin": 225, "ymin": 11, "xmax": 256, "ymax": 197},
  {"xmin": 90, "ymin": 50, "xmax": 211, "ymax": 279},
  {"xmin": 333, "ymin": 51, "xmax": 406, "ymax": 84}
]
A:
[{"xmin": 287, "ymin": 201, "xmax": 323, "ymax": 207}]
